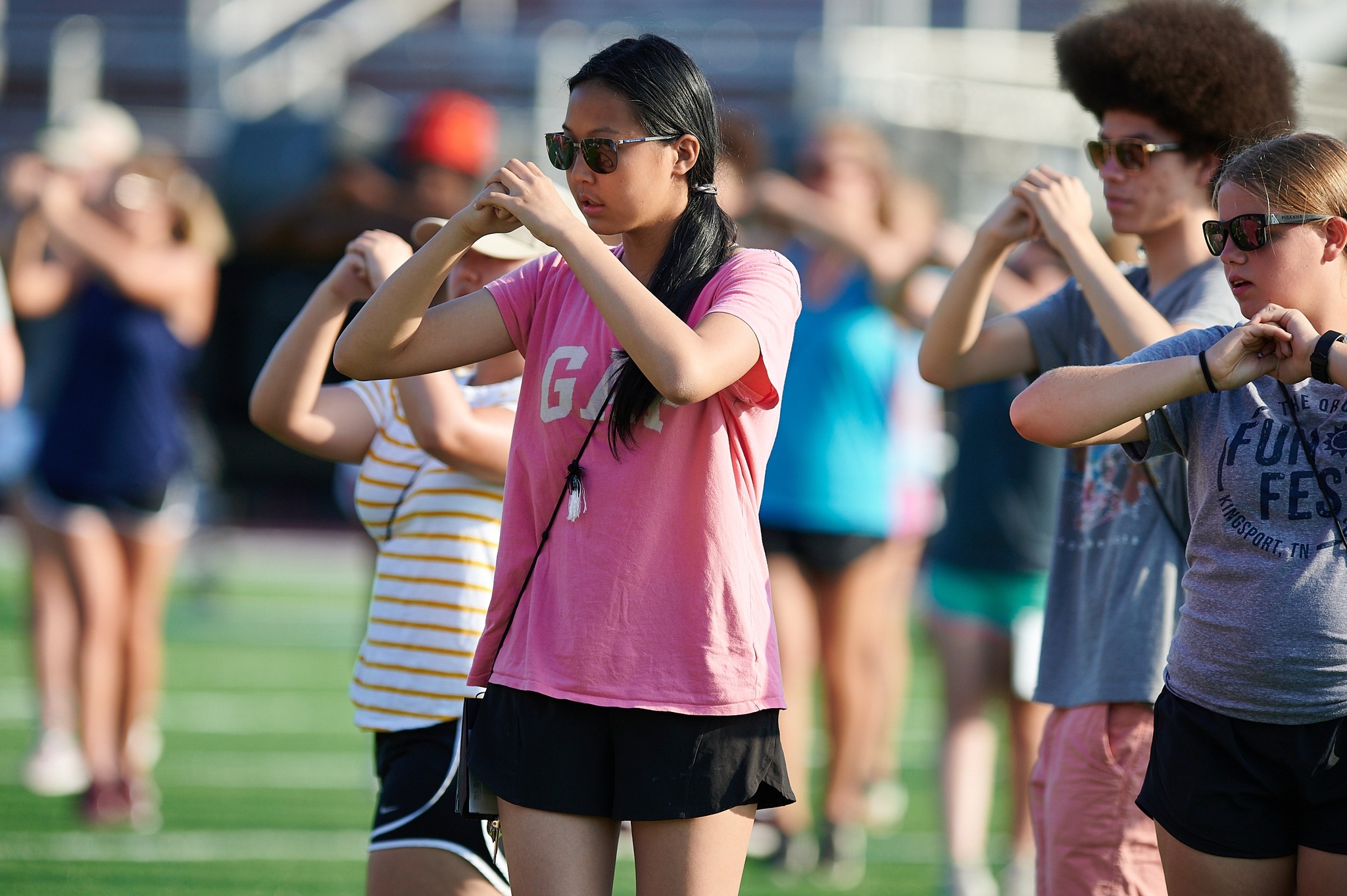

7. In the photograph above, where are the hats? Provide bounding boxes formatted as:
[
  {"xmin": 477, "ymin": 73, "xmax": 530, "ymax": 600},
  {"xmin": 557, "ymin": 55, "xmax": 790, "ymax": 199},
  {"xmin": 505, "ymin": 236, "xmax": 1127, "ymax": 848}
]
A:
[
  {"xmin": 368, "ymin": 87, "xmax": 495, "ymax": 176},
  {"xmin": 29, "ymin": 97, "xmax": 140, "ymax": 166}
]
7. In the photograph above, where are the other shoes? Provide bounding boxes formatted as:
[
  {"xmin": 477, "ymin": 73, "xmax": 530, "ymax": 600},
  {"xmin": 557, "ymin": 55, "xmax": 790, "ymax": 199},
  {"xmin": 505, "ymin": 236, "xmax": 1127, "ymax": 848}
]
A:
[{"xmin": 20, "ymin": 729, "xmax": 160, "ymax": 822}]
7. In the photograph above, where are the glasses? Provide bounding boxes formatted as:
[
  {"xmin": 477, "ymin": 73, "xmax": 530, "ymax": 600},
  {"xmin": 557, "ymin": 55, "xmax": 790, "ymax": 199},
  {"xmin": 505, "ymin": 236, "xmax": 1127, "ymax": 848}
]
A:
[
  {"xmin": 1087, "ymin": 138, "xmax": 1187, "ymax": 172},
  {"xmin": 1202, "ymin": 214, "xmax": 1347, "ymax": 258},
  {"xmin": 545, "ymin": 132, "xmax": 680, "ymax": 177}
]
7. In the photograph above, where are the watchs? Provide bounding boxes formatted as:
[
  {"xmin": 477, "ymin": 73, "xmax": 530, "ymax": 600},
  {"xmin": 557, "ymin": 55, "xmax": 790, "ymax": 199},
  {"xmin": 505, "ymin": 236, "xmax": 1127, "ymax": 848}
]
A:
[{"xmin": 1308, "ymin": 329, "xmax": 1347, "ymax": 384}]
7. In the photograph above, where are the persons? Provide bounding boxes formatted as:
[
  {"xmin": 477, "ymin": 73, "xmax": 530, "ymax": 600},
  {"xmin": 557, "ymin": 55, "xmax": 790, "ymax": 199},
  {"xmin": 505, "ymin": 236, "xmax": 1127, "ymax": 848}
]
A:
[
  {"xmin": 0, "ymin": 101, "xmax": 233, "ymax": 833},
  {"xmin": 1010, "ymin": 135, "xmax": 1347, "ymax": 896},
  {"xmin": 332, "ymin": 91, "xmax": 493, "ymax": 517},
  {"xmin": 696, "ymin": 120, "xmax": 1147, "ymax": 895},
  {"xmin": 919, "ymin": 2, "xmax": 1302, "ymax": 896},
  {"xmin": 251, "ymin": 213, "xmax": 557, "ymax": 896},
  {"xmin": 332, "ymin": 34, "xmax": 802, "ymax": 896}
]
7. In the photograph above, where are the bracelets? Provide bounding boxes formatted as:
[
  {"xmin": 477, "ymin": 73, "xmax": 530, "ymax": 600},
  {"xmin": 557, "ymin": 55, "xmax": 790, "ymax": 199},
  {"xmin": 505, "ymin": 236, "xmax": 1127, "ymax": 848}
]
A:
[{"xmin": 1198, "ymin": 350, "xmax": 1218, "ymax": 395}]
